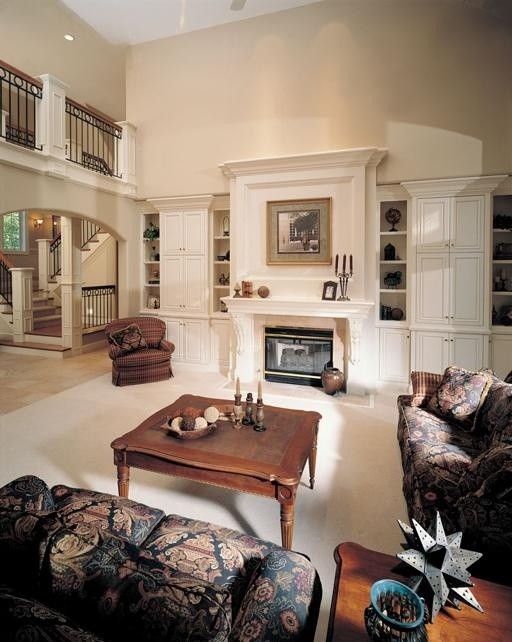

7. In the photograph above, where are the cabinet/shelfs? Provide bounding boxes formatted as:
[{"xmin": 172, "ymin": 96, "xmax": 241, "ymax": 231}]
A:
[
  {"xmin": 139, "ymin": 212, "xmax": 160, "ymax": 311},
  {"xmin": 164, "ymin": 318, "xmax": 207, "ymax": 362},
  {"xmin": 211, "ymin": 319, "xmax": 229, "ymax": 364},
  {"xmin": 490, "ymin": 194, "xmax": 511, "ymax": 327},
  {"xmin": 379, "ymin": 326, "xmax": 409, "ymax": 381},
  {"xmin": 210, "ymin": 209, "xmax": 230, "ymax": 314},
  {"xmin": 160, "ymin": 209, "xmax": 207, "ymax": 314},
  {"xmin": 414, "ymin": 194, "xmax": 487, "ymax": 327},
  {"xmin": 378, "ymin": 198, "xmax": 410, "ymax": 323},
  {"xmin": 65, "ymin": 139, "xmax": 81, "ymax": 163},
  {"xmin": 491, "ymin": 334, "xmax": 511, "ymax": 381},
  {"xmin": 411, "ymin": 331, "xmax": 485, "ymax": 375}
]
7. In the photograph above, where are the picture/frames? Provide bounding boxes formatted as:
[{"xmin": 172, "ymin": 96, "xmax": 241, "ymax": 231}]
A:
[
  {"xmin": 266, "ymin": 197, "xmax": 332, "ymax": 265},
  {"xmin": 322, "ymin": 281, "xmax": 338, "ymax": 301}
]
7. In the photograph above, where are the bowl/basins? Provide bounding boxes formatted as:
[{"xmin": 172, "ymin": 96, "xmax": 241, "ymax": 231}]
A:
[{"xmin": 217, "ymin": 256, "xmax": 225, "ymax": 261}]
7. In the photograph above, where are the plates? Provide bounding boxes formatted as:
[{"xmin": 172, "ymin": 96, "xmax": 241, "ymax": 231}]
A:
[{"xmin": 392, "ymin": 308, "xmax": 403, "ymax": 320}]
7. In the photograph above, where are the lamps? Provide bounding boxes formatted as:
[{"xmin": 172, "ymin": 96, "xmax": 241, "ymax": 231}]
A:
[{"xmin": 33, "ymin": 217, "xmax": 44, "ymax": 234}]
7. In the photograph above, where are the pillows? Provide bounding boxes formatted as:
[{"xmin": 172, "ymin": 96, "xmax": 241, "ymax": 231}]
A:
[
  {"xmin": 426, "ymin": 366, "xmax": 493, "ymax": 433},
  {"xmin": 410, "ymin": 371, "xmax": 444, "ymax": 407},
  {"xmin": 110, "ymin": 323, "xmax": 148, "ymax": 354}
]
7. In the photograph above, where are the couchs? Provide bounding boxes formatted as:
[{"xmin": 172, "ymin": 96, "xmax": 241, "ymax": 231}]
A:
[
  {"xmin": 0, "ymin": 475, "xmax": 322, "ymax": 642},
  {"xmin": 104, "ymin": 317, "xmax": 175, "ymax": 387},
  {"xmin": 397, "ymin": 375, "xmax": 511, "ymax": 548}
]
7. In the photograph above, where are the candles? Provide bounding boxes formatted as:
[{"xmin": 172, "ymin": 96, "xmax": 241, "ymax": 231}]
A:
[
  {"xmin": 335, "ymin": 253, "xmax": 339, "ymax": 273},
  {"xmin": 350, "ymin": 254, "xmax": 353, "ymax": 276},
  {"xmin": 342, "ymin": 254, "xmax": 347, "ymax": 273},
  {"xmin": 236, "ymin": 376, "xmax": 241, "ymax": 396},
  {"xmin": 257, "ymin": 377, "xmax": 262, "ymax": 400}
]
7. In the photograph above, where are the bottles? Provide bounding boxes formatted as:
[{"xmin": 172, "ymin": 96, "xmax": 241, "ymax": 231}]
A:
[
  {"xmin": 219, "ymin": 273, "xmax": 227, "ymax": 285},
  {"xmin": 151, "ymin": 247, "xmax": 156, "ymax": 261},
  {"xmin": 493, "ymin": 305, "xmax": 497, "ymax": 325}
]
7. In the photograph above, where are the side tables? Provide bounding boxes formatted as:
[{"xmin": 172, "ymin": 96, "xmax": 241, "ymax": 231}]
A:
[{"xmin": 326, "ymin": 542, "xmax": 511, "ymax": 642}]
